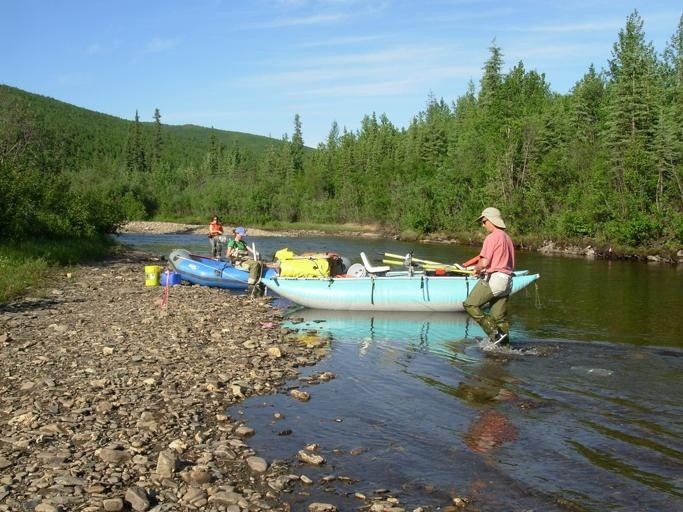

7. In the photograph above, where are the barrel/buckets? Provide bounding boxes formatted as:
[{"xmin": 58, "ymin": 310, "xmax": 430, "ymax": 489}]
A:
[
  {"xmin": 347, "ymin": 263, "xmax": 367, "ymax": 278},
  {"xmin": 144, "ymin": 265, "xmax": 160, "ymax": 286}
]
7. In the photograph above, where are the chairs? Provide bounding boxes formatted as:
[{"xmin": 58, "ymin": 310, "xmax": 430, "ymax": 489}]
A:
[{"xmin": 359, "ymin": 252, "xmax": 389, "ymax": 273}]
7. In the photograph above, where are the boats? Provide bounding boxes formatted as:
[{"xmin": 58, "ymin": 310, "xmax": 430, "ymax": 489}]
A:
[
  {"xmin": 259, "ymin": 269, "xmax": 541, "ymax": 312},
  {"xmin": 168, "ymin": 247, "xmax": 279, "ymax": 290}
]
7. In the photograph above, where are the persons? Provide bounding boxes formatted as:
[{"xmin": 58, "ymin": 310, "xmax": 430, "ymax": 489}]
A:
[
  {"xmin": 209, "ymin": 215, "xmax": 225, "ymax": 259},
  {"xmin": 227, "ymin": 227, "xmax": 260, "ymax": 291},
  {"xmin": 406, "ymin": 248, "xmax": 415, "ymax": 276},
  {"xmin": 330, "ymin": 256, "xmax": 348, "ymax": 279},
  {"xmin": 463, "ymin": 208, "xmax": 514, "ymax": 348}
]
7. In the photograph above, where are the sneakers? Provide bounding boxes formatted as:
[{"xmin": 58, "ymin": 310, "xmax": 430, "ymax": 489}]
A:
[
  {"xmin": 248, "ymin": 284, "xmax": 255, "ymax": 290},
  {"xmin": 483, "ymin": 329, "xmax": 507, "ymax": 352}
]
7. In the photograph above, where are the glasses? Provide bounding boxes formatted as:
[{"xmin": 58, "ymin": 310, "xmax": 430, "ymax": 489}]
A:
[{"xmin": 483, "ymin": 219, "xmax": 488, "ymax": 224}]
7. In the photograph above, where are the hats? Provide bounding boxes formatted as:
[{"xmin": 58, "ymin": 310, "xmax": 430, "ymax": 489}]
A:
[
  {"xmin": 235, "ymin": 227, "xmax": 247, "ymax": 236},
  {"xmin": 470, "ymin": 207, "xmax": 507, "ymax": 229}
]
